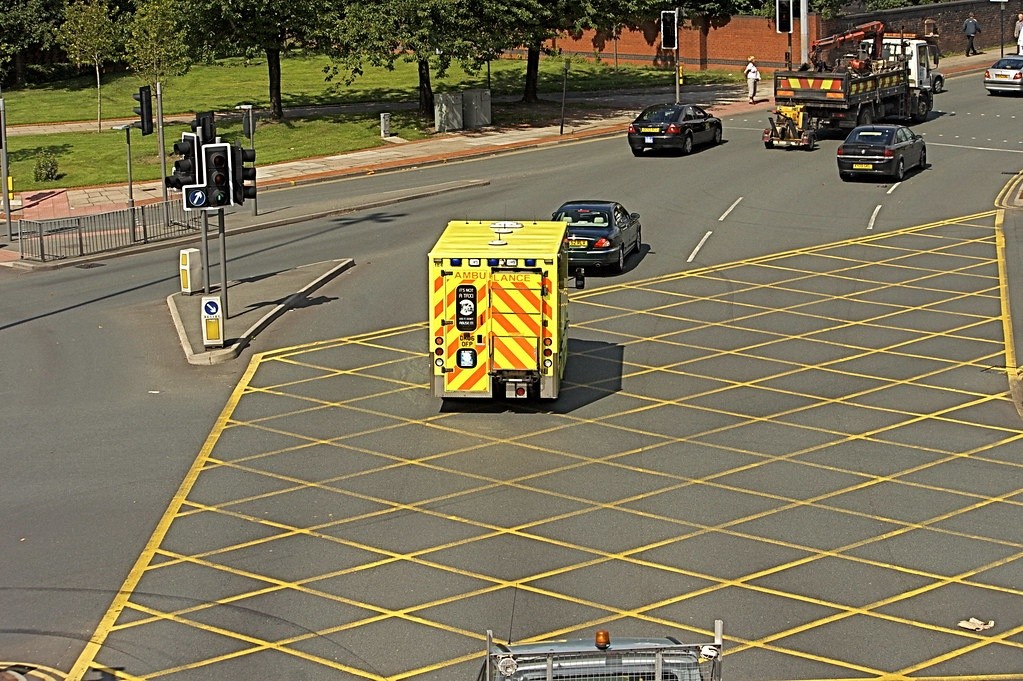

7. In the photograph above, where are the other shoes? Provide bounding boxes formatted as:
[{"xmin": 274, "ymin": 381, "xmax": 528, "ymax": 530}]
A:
[
  {"xmin": 1016, "ymin": 51, "xmax": 1020, "ymax": 55},
  {"xmin": 749, "ymin": 99, "xmax": 753, "ymax": 104},
  {"xmin": 966, "ymin": 53, "xmax": 970, "ymax": 57},
  {"xmin": 973, "ymin": 51, "xmax": 977, "ymax": 55}
]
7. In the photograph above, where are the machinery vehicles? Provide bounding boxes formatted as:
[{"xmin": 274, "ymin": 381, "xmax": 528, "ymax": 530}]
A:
[
  {"xmin": 773, "ymin": 20, "xmax": 940, "ymax": 139},
  {"xmin": 761, "ymin": 104, "xmax": 817, "ymax": 149}
]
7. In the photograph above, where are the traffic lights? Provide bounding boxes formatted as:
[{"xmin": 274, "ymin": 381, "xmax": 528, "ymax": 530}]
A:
[
  {"xmin": 202, "ymin": 143, "xmax": 233, "ymax": 209},
  {"xmin": 776, "ymin": 0, "xmax": 794, "ymax": 33},
  {"xmin": 660, "ymin": 10, "xmax": 681, "ymax": 50},
  {"xmin": 234, "ymin": 146, "xmax": 259, "ymax": 206},
  {"xmin": 190, "ymin": 110, "xmax": 216, "ymax": 143},
  {"xmin": 133, "ymin": 86, "xmax": 154, "ymax": 135},
  {"xmin": 164, "ymin": 132, "xmax": 199, "ymax": 192}
]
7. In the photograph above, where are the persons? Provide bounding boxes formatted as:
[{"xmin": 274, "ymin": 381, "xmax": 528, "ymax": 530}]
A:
[
  {"xmin": 744, "ymin": 55, "xmax": 762, "ymax": 104},
  {"xmin": 1014, "ymin": 14, "xmax": 1023, "ymax": 54},
  {"xmin": 962, "ymin": 12, "xmax": 982, "ymax": 57}
]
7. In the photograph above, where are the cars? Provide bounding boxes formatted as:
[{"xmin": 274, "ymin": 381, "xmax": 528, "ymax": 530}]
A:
[
  {"xmin": 838, "ymin": 125, "xmax": 927, "ymax": 181},
  {"xmin": 930, "ymin": 70, "xmax": 945, "ymax": 93},
  {"xmin": 982, "ymin": 56, "xmax": 1023, "ymax": 94},
  {"xmin": 627, "ymin": 104, "xmax": 723, "ymax": 156},
  {"xmin": 551, "ymin": 199, "xmax": 642, "ymax": 274}
]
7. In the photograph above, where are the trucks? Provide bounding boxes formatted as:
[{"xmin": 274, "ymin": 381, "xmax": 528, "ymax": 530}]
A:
[{"xmin": 424, "ymin": 222, "xmax": 586, "ymax": 404}]
